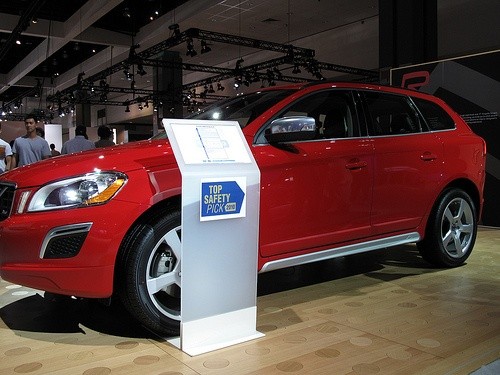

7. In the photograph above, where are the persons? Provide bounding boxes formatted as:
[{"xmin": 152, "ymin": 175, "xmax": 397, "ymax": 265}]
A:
[
  {"xmin": 95, "ymin": 125, "xmax": 116, "ymax": 149},
  {"xmin": 10, "ymin": 114, "xmax": 52, "ymax": 170},
  {"xmin": 61, "ymin": 125, "xmax": 96, "ymax": 154},
  {"xmin": 0, "ymin": 122, "xmax": 13, "ymax": 175},
  {"xmin": 35, "ymin": 127, "xmax": 45, "ymax": 138},
  {"xmin": 50, "ymin": 144, "xmax": 60, "ymax": 157}
]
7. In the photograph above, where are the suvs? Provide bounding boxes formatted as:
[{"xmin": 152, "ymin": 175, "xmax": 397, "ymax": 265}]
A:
[{"xmin": 0, "ymin": 80, "xmax": 488, "ymax": 339}]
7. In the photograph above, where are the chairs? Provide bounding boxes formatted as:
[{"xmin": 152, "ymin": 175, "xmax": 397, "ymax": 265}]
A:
[
  {"xmin": 390, "ymin": 113, "xmax": 412, "ymax": 133},
  {"xmin": 322, "ymin": 108, "xmax": 347, "ymax": 138}
]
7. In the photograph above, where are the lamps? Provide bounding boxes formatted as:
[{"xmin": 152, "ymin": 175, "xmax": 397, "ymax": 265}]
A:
[{"xmin": 2, "ymin": 9, "xmax": 327, "ymax": 115}]
